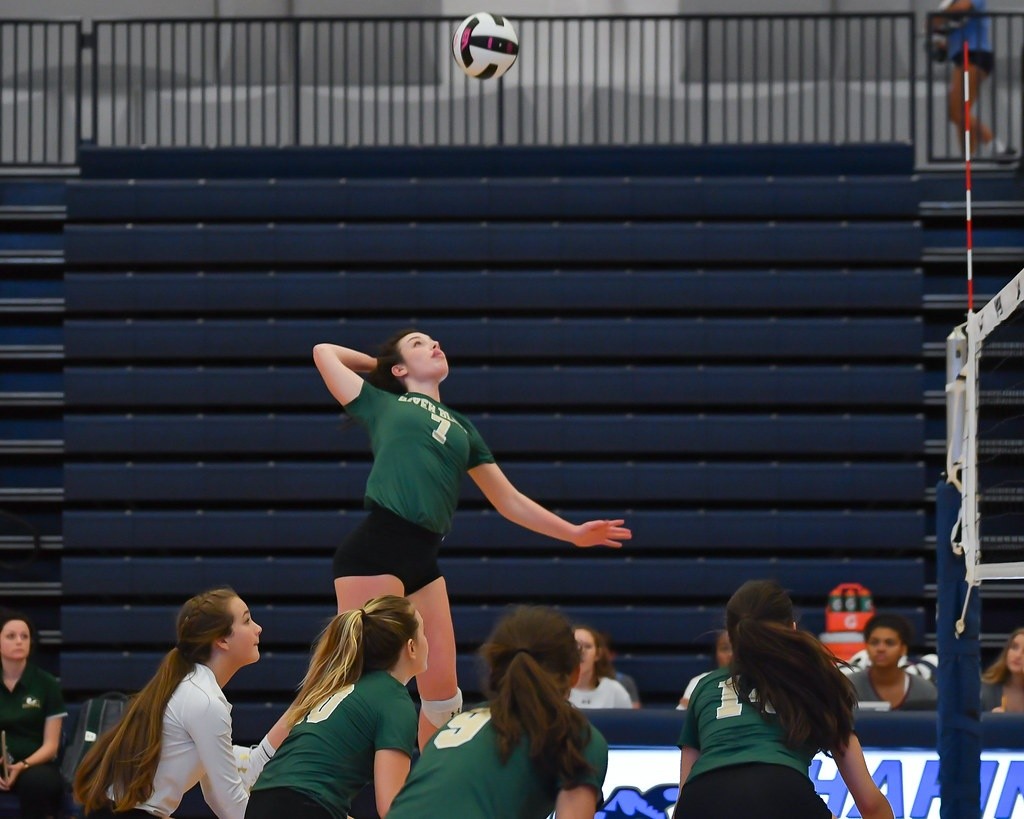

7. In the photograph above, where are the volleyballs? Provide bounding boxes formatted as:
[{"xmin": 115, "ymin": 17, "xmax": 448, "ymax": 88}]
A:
[{"xmin": 452, "ymin": 11, "xmax": 519, "ymax": 80}]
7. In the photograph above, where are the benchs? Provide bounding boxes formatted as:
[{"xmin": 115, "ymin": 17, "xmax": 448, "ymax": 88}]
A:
[{"xmin": 0, "ymin": 137, "xmax": 1024, "ymax": 743}]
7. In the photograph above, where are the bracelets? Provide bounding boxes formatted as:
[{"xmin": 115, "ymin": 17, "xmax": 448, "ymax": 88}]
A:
[{"xmin": 21, "ymin": 760, "xmax": 31, "ymax": 769}]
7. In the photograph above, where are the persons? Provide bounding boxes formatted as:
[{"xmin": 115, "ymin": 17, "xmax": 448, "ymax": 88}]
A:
[
  {"xmin": 845, "ymin": 614, "xmax": 935, "ymax": 707},
  {"xmin": 567, "ymin": 626, "xmax": 732, "ymax": 711},
  {"xmin": 978, "ymin": 627, "xmax": 1024, "ymax": 716},
  {"xmin": 384, "ymin": 607, "xmax": 608, "ymax": 819},
  {"xmin": 73, "ymin": 587, "xmax": 299, "ymax": 819},
  {"xmin": 924, "ymin": 0, "xmax": 1018, "ymax": 164},
  {"xmin": 670, "ymin": 581, "xmax": 895, "ymax": 819},
  {"xmin": 244, "ymin": 595, "xmax": 428, "ymax": 819},
  {"xmin": 314, "ymin": 330, "xmax": 632, "ymax": 753},
  {"xmin": 0, "ymin": 612, "xmax": 71, "ymax": 819}
]
